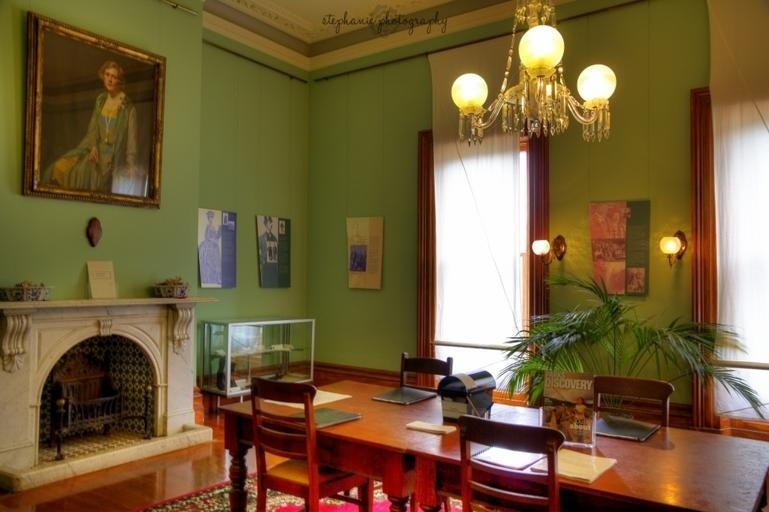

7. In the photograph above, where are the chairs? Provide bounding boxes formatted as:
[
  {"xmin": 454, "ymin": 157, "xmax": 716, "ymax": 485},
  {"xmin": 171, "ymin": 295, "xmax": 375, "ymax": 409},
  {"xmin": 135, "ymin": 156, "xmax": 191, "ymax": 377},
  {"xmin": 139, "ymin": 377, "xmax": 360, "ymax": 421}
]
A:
[
  {"xmin": 398, "ymin": 351, "xmax": 455, "ymax": 391},
  {"xmin": 242, "ymin": 372, "xmax": 378, "ymax": 512},
  {"xmin": 593, "ymin": 375, "xmax": 674, "ymax": 428},
  {"xmin": 450, "ymin": 413, "xmax": 568, "ymax": 512}
]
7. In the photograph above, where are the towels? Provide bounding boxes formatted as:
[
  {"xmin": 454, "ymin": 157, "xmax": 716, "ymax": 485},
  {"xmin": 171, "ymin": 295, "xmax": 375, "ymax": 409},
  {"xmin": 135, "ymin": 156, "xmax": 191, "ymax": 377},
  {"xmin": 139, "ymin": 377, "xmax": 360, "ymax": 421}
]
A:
[{"xmin": 405, "ymin": 420, "xmax": 458, "ymax": 436}]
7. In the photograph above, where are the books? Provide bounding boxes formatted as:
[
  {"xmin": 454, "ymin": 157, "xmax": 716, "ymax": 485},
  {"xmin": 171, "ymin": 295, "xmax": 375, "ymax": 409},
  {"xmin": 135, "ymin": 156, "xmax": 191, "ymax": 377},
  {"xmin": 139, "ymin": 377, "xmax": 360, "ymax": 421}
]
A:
[
  {"xmin": 371, "ymin": 384, "xmax": 437, "ymax": 405},
  {"xmin": 596, "ymin": 413, "xmax": 661, "ymax": 443},
  {"xmin": 87, "ymin": 258, "xmax": 117, "ymax": 300},
  {"xmin": 472, "ymin": 435, "xmax": 621, "ymax": 485},
  {"xmin": 543, "ymin": 371, "xmax": 592, "ymax": 443},
  {"xmin": 293, "ymin": 405, "xmax": 359, "ymax": 432}
]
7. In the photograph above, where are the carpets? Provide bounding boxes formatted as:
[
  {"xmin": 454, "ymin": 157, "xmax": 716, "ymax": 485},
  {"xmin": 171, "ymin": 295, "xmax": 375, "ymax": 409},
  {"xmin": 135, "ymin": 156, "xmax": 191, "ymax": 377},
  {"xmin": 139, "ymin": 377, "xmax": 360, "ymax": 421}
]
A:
[{"xmin": 131, "ymin": 468, "xmax": 493, "ymax": 512}]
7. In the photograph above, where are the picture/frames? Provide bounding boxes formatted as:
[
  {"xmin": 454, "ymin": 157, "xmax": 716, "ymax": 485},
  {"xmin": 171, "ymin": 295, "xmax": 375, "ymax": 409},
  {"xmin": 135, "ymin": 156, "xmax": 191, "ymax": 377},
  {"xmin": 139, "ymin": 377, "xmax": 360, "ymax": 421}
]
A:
[{"xmin": 18, "ymin": 7, "xmax": 168, "ymax": 210}]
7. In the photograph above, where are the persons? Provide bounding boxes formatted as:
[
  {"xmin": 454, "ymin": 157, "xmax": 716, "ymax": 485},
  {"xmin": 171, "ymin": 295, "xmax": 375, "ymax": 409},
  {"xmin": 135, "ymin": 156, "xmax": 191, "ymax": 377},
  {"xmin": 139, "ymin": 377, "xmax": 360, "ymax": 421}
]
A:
[
  {"xmin": 258, "ymin": 215, "xmax": 279, "ymax": 288},
  {"xmin": 574, "ymin": 397, "xmax": 590, "ymax": 442},
  {"xmin": 200, "ymin": 211, "xmax": 222, "ymax": 285},
  {"xmin": 44, "ymin": 60, "xmax": 139, "ymax": 194}
]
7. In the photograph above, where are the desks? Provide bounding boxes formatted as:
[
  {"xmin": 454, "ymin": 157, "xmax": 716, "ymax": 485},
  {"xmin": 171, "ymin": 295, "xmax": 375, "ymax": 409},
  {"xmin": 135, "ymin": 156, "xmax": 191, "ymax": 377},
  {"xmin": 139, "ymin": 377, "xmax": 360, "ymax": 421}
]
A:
[{"xmin": 218, "ymin": 376, "xmax": 768, "ymax": 511}]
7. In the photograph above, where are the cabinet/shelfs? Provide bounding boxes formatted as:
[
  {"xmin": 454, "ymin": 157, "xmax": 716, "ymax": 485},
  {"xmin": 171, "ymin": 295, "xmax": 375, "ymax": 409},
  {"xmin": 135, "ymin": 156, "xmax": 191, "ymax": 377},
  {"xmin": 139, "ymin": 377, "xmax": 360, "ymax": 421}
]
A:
[{"xmin": 199, "ymin": 317, "xmax": 318, "ymax": 400}]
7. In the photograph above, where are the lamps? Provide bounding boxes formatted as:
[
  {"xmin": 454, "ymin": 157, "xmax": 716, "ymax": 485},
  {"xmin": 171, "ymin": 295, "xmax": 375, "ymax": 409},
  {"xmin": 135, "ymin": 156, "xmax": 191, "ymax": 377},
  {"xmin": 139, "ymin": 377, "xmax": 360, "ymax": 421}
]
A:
[
  {"xmin": 450, "ymin": 1, "xmax": 618, "ymax": 151},
  {"xmin": 657, "ymin": 229, "xmax": 689, "ymax": 270},
  {"xmin": 532, "ymin": 234, "xmax": 569, "ymax": 267}
]
